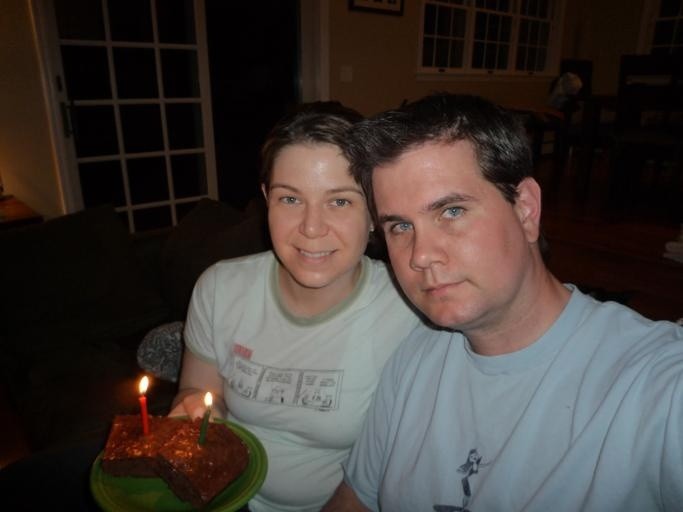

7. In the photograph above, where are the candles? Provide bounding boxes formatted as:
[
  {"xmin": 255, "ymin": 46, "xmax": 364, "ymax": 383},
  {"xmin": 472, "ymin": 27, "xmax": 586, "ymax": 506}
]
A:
[
  {"xmin": 132, "ymin": 374, "xmax": 153, "ymax": 436},
  {"xmin": 196, "ymin": 390, "xmax": 215, "ymax": 446}
]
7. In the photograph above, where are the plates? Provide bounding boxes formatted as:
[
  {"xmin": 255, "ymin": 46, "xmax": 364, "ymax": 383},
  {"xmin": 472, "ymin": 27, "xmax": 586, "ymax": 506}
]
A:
[{"xmin": 92, "ymin": 415, "xmax": 269, "ymax": 510}]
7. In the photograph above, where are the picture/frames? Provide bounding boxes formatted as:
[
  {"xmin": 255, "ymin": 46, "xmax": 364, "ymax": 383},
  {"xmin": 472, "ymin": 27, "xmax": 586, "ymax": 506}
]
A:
[{"xmin": 348, "ymin": 0, "xmax": 407, "ymax": 16}]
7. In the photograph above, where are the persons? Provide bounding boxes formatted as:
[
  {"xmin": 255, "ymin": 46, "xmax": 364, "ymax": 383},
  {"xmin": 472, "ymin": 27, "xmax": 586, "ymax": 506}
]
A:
[
  {"xmin": 311, "ymin": 91, "xmax": 683, "ymax": 512},
  {"xmin": 163, "ymin": 102, "xmax": 434, "ymax": 511}
]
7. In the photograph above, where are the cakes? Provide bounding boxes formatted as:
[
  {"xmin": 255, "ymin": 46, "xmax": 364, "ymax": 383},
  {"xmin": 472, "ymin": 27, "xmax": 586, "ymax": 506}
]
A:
[
  {"xmin": 102, "ymin": 415, "xmax": 186, "ymax": 477},
  {"xmin": 158, "ymin": 417, "xmax": 249, "ymax": 508}
]
